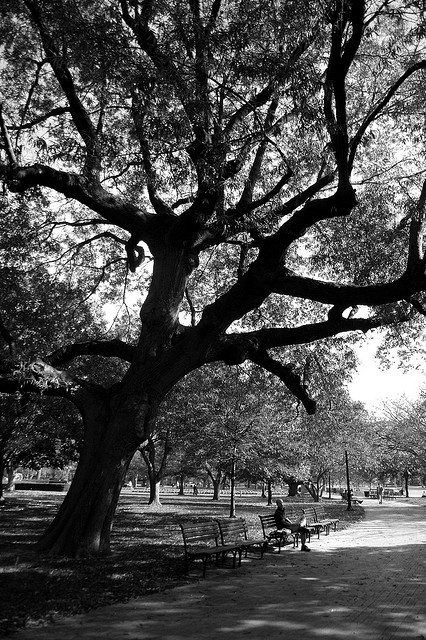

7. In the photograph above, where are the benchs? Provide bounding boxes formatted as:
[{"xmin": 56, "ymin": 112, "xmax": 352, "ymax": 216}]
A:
[
  {"xmin": 217, "ymin": 517, "xmax": 266, "ymax": 568},
  {"xmin": 301, "ymin": 507, "xmax": 331, "ymax": 539},
  {"xmin": 312, "ymin": 506, "xmax": 339, "ymax": 534},
  {"xmin": 290, "ymin": 511, "xmax": 315, "ymax": 547},
  {"xmin": 258, "ymin": 514, "xmax": 295, "ymax": 554},
  {"xmin": 180, "ymin": 522, "xmax": 239, "ymax": 578}
]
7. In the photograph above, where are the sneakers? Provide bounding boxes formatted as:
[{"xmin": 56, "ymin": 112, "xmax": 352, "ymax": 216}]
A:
[{"xmin": 301, "ymin": 545, "xmax": 310, "ymax": 551}]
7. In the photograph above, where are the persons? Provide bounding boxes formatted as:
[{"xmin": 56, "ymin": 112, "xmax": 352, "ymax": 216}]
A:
[
  {"xmin": 377, "ymin": 484, "xmax": 383, "ymax": 504},
  {"xmin": 275, "ymin": 499, "xmax": 311, "ymax": 551},
  {"xmin": 345, "ymin": 490, "xmax": 363, "ymax": 504}
]
